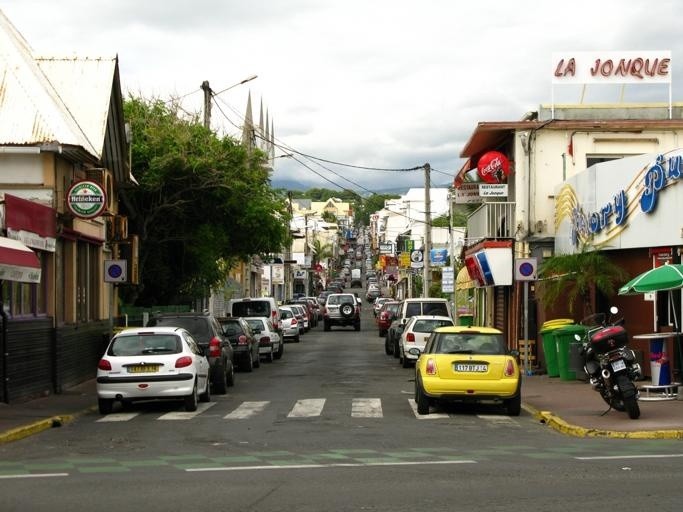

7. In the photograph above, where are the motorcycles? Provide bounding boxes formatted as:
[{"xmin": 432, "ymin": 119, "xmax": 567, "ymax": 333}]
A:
[{"xmin": 572, "ymin": 304, "xmax": 643, "ymax": 418}]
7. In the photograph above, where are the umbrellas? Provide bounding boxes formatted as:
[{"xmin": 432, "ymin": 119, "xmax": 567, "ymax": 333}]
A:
[{"xmin": 615, "ymin": 262, "xmax": 683, "ymax": 333}]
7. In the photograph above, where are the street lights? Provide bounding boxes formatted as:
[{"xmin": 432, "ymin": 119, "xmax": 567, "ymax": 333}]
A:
[
  {"xmin": 254, "ymin": 152, "xmax": 297, "ymax": 165},
  {"xmin": 198, "ymin": 71, "xmax": 261, "ymax": 142}
]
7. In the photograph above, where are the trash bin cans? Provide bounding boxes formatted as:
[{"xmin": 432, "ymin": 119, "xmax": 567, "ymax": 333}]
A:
[{"xmin": 538, "ymin": 319, "xmax": 589, "ymax": 381}]
